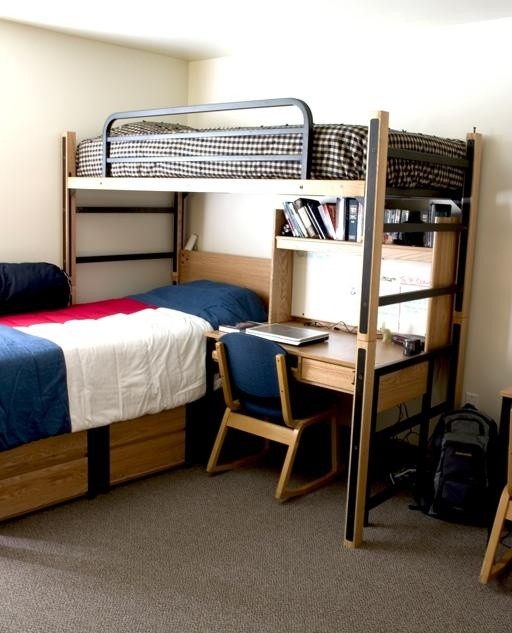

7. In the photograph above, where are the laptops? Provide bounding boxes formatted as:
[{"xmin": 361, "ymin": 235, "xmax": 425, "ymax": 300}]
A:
[{"xmin": 244, "ymin": 322, "xmax": 330, "ymax": 347}]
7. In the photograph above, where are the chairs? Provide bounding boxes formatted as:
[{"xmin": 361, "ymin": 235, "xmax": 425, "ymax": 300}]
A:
[
  {"xmin": 206, "ymin": 332, "xmax": 347, "ymax": 502},
  {"xmin": 476, "ymin": 387, "xmax": 512, "ymax": 585}
]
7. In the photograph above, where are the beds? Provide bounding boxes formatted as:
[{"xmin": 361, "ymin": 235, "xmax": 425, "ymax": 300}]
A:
[
  {"xmin": 0, "ymin": 249, "xmax": 272, "ymax": 525},
  {"xmin": 60, "ymin": 98, "xmax": 483, "ymax": 197}
]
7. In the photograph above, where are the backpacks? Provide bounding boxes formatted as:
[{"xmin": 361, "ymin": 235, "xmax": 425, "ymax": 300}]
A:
[{"xmin": 412, "ymin": 402, "xmax": 498, "ymax": 528}]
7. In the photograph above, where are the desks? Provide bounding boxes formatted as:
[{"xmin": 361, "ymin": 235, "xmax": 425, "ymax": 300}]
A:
[{"xmin": 208, "ymin": 316, "xmax": 426, "ymax": 547}]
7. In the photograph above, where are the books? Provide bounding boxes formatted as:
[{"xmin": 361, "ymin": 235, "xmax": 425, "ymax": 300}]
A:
[{"xmin": 280, "ymin": 195, "xmax": 453, "ymax": 248}]
